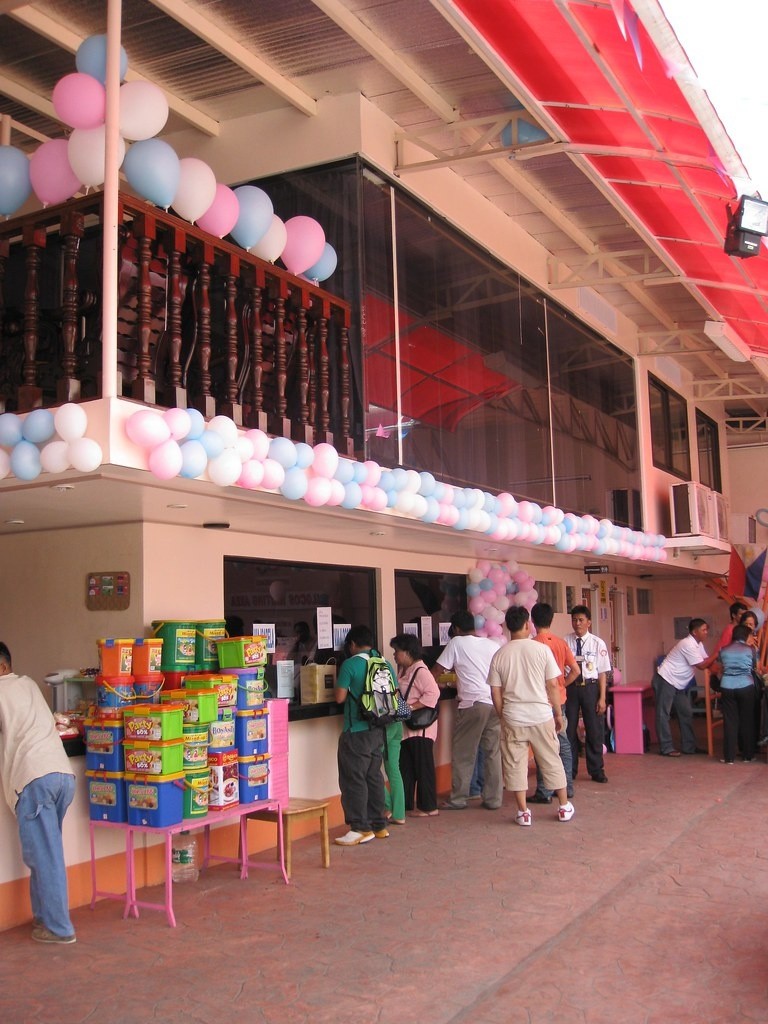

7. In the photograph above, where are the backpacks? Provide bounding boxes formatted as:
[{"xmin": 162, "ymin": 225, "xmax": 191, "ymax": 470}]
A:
[{"xmin": 347, "ymin": 653, "xmax": 398, "ymax": 728}]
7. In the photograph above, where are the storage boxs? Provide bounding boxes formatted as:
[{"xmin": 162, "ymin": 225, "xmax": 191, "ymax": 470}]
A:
[
  {"xmin": 122, "ymin": 702, "xmax": 185, "ymax": 742},
  {"xmin": 215, "ymin": 635, "xmax": 268, "ymax": 669},
  {"xmin": 122, "ymin": 738, "xmax": 185, "ymax": 775},
  {"xmin": 238, "ymin": 753, "xmax": 272, "ymax": 803},
  {"xmin": 83, "ymin": 719, "xmax": 125, "ymax": 773},
  {"xmin": 84, "ymin": 770, "xmax": 128, "ymax": 824},
  {"xmin": 157, "ymin": 665, "xmax": 214, "ymax": 690},
  {"xmin": 124, "ymin": 771, "xmax": 186, "ymax": 827},
  {"xmin": 160, "ymin": 688, "xmax": 219, "ymax": 724},
  {"xmin": 184, "ymin": 673, "xmax": 240, "ymax": 707},
  {"xmin": 235, "ymin": 708, "xmax": 270, "ymax": 757}
]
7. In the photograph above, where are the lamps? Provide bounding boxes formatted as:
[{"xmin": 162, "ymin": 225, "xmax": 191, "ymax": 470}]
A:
[
  {"xmin": 703, "ymin": 321, "xmax": 752, "ymax": 363},
  {"xmin": 482, "ymin": 352, "xmax": 546, "ymax": 390},
  {"xmin": 723, "ymin": 194, "xmax": 768, "ymax": 260}
]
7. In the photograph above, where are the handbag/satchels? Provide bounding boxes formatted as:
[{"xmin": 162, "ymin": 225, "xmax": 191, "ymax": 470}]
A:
[
  {"xmin": 404, "ymin": 667, "xmax": 439, "ymax": 730},
  {"xmin": 395, "ymin": 688, "xmax": 411, "ymax": 722},
  {"xmin": 752, "ymin": 670, "xmax": 766, "ymax": 698},
  {"xmin": 299, "ymin": 656, "xmax": 337, "ymax": 706}
]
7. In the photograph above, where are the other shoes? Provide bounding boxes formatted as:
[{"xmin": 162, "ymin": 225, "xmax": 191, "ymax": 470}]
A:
[
  {"xmin": 438, "ymin": 801, "xmax": 468, "ymax": 809},
  {"xmin": 384, "ymin": 811, "xmax": 405, "ymax": 825},
  {"xmin": 335, "ymin": 829, "xmax": 374, "ymax": 846},
  {"xmin": 526, "ymin": 794, "xmax": 553, "ymax": 803},
  {"xmin": 667, "ymin": 751, "xmax": 681, "ymax": 757},
  {"xmin": 683, "ymin": 749, "xmax": 707, "ymax": 755},
  {"xmin": 407, "ymin": 809, "xmax": 439, "ymax": 817},
  {"xmin": 719, "ymin": 757, "xmax": 734, "ymax": 764},
  {"xmin": 567, "ymin": 794, "xmax": 574, "ymax": 798},
  {"xmin": 591, "ymin": 775, "xmax": 608, "ymax": 783},
  {"xmin": 742, "ymin": 756, "xmax": 755, "ymax": 763},
  {"xmin": 375, "ymin": 830, "xmax": 389, "ymax": 837},
  {"xmin": 468, "ymin": 792, "xmax": 480, "ymax": 799}
]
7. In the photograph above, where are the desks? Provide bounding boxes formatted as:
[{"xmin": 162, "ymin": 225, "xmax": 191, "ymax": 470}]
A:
[
  {"xmin": 89, "ymin": 798, "xmax": 292, "ymax": 927},
  {"xmin": 609, "ymin": 679, "xmax": 656, "ymax": 755},
  {"xmin": 0, "ymin": 219, "xmax": 350, "ymax": 454},
  {"xmin": 237, "ymin": 797, "xmax": 332, "ymax": 879}
]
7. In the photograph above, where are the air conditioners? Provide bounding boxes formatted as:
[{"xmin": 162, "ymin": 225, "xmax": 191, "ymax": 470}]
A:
[
  {"xmin": 670, "ymin": 481, "xmax": 715, "ymax": 538},
  {"xmin": 730, "ymin": 513, "xmax": 757, "ymax": 544},
  {"xmin": 711, "ymin": 491, "xmax": 731, "ymax": 543}
]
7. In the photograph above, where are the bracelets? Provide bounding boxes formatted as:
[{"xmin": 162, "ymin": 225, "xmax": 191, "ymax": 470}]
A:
[{"xmin": 410, "ymin": 704, "xmax": 414, "ymax": 711}]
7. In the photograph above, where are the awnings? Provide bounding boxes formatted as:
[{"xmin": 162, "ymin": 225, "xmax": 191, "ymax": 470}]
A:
[
  {"xmin": 449, "ymin": 0, "xmax": 768, "ymax": 355},
  {"xmin": 363, "ymin": 289, "xmax": 522, "ymax": 434}
]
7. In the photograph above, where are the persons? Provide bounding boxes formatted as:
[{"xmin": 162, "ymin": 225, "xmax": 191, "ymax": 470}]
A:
[
  {"xmin": 0, "ymin": 641, "xmax": 77, "ymax": 944},
  {"xmin": 389, "ymin": 633, "xmax": 440, "ymax": 817},
  {"xmin": 526, "ymin": 602, "xmax": 581, "ymax": 804},
  {"xmin": 713, "ymin": 602, "xmax": 768, "ymax": 765},
  {"xmin": 563, "ymin": 605, "xmax": 611, "ymax": 784},
  {"xmin": 285, "ymin": 620, "xmax": 319, "ymax": 704},
  {"xmin": 431, "ymin": 611, "xmax": 502, "ymax": 810},
  {"xmin": 485, "ymin": 605, "xmax": 574, "ymax": 825},
  {"xmin": 651, "ymin": 617, "xmax": 718, "ymax": 757},
  {"xmin": 334, "ymin": 624, "xmax": 406, "ymax": 845}
]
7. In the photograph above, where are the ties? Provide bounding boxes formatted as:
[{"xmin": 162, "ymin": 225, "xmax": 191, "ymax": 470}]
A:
[{"xmin": 574, "ymin": 638, "xmax": 583, "ymax": 684}]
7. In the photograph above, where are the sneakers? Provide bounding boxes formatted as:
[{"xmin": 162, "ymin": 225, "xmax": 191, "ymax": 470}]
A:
[
  {"xmin": 558, "ymin": 801, "xmax": 574, "ymax": 821},
  {"xmin": 514, "ymin": 808, "xmax": 531, "ymax": 826},
  {"xmin": 31, "ymin": 917, "xmax": 76, "ymax": 944}
]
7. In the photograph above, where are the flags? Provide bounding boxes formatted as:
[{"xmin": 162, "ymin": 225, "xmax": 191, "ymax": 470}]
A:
[{"xmin": 728, "ymin": 543, "xmax": 767, "ymax": 603}]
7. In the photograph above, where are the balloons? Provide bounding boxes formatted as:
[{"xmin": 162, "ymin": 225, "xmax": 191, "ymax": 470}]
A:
[
  {"xmin": 0, "ymin": 403, "xmax": 667, "ymax": 561},
  {"xmin": 748, "ymin": 607, "xmax": 765, "ymax": 633},
  {"xmin": 0, "ymin": 32, "xmax": 337, "ymax": 284},
  {"xmin": 466, "ymin": 560, "xmax": 538, "ymax": 646}
]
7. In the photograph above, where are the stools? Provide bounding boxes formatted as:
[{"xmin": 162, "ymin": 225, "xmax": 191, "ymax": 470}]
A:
[{"xmin": 688, "ymin": 685, "xmax": 714, "ymax": 718}]
